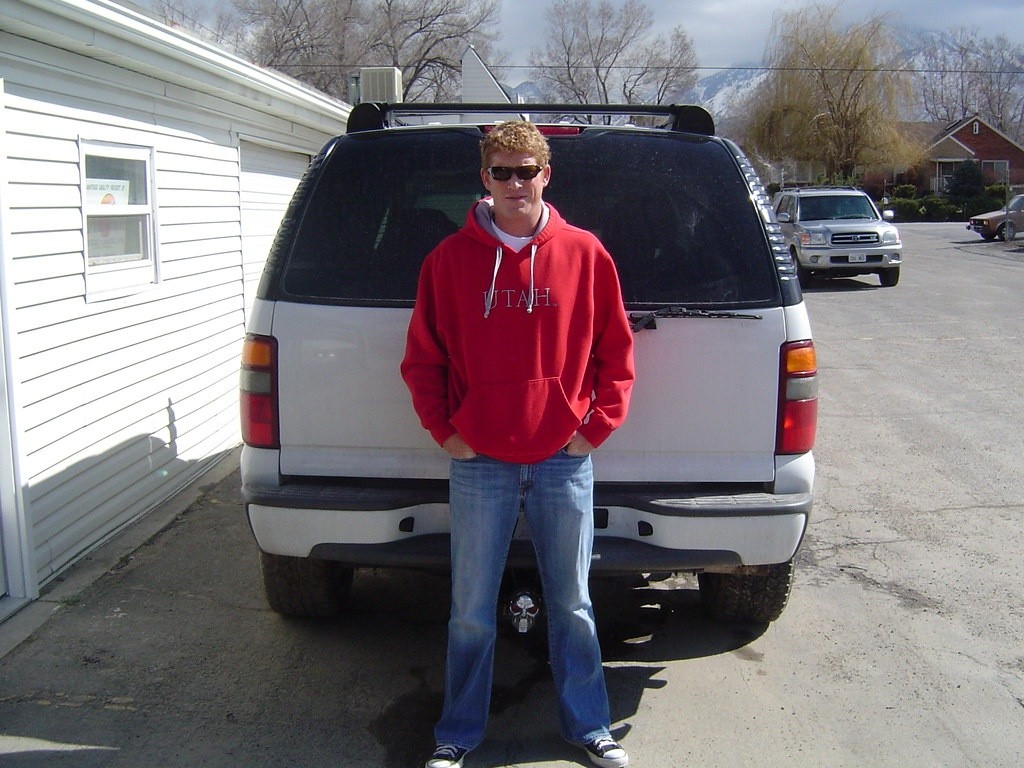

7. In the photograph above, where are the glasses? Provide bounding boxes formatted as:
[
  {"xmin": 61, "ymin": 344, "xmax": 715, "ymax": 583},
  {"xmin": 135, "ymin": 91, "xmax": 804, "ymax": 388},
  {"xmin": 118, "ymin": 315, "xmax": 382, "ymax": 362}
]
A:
[{"xmin": 486, "ymin": 164, "xmax": 543, "ymax": 181}]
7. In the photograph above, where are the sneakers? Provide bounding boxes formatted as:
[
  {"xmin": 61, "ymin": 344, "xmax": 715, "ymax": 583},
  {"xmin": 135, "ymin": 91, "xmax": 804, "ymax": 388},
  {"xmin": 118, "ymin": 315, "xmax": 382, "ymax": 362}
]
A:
[
  {"xmin": 425, "ymin": 735, "xmax": 485, "ymax": 768},
  {"xmin": 560, "ymin": 726, "xmax": 629, "ymax": 768}
]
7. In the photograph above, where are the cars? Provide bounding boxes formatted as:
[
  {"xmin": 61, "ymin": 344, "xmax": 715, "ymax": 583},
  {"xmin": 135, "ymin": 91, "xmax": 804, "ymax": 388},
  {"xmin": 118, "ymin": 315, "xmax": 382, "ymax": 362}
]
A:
[{"xmin": 967, "ymin": 194, "xmax": 1024, "ymax": 241}]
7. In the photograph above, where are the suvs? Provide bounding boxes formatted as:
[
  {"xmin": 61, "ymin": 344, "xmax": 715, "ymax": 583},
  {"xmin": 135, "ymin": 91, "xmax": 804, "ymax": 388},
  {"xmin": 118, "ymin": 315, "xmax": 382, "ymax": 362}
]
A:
[
  {"xmin": 772, "ymin": 188, "xmax": 903, "ymax": 288},
  {"xmin": 239, "ymin": 98, "xmax": 821, "ymax": 626}
]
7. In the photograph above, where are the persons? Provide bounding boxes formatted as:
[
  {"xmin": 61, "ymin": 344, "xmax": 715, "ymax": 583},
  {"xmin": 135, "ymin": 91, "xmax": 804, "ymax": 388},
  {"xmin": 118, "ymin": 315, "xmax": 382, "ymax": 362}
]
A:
[{"xmin": 399, "ymin": 116, "xmax": 637, "ymax": 768}]
